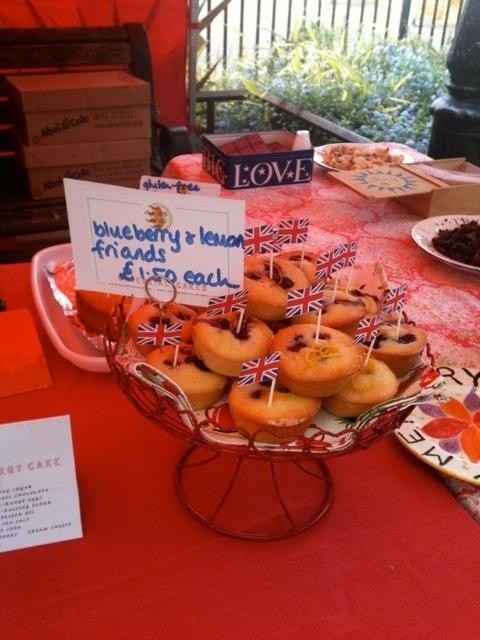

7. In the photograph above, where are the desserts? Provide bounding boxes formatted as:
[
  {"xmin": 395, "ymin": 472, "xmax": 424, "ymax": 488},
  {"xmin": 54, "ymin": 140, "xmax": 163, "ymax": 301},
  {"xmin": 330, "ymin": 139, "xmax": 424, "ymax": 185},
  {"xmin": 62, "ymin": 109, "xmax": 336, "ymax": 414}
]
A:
[{"xmin": 127, "ymin": 244, "xmax": 432, "ymax": 447}]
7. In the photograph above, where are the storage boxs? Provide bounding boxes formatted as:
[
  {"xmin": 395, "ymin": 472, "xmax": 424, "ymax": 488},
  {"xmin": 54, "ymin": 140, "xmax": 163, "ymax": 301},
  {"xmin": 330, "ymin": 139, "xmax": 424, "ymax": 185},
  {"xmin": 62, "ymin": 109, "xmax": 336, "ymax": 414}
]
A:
[
  {"xmin": 8, "ymin": 71, "xmax": 153, "ymax": 139},
  {"xmin": 19, "ymin": 139, "xmax": 150, "ymax": 197},
  {"xmin": 328, "ymin": 156, "xmax": 480, "ymax": 216},
  {"xmin": 201, "ymin": 126, "xmax": 314, "ymax": 189}
]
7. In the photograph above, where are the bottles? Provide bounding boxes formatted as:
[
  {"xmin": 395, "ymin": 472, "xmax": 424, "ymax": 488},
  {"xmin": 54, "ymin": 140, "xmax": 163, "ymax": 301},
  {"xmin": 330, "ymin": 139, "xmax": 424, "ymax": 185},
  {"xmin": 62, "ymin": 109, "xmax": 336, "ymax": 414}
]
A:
[{"xmin": 292, "ymin": 130, "xmax": 311, "ymax": 152}]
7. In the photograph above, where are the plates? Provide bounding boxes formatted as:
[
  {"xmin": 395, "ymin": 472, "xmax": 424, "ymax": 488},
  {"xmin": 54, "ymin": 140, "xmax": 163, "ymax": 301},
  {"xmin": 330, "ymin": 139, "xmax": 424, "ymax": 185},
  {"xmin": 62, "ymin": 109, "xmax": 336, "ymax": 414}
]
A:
[
  {"xmin": 313, "ymin": 142, "xmax": 413, "ymax": 172},
  {"xmin": 394, "ymin": 367, "xmax": 480, "ymax": 486},
  {"xmin": 412, "ymin": 215, "xmax": 480, "ymax": 276},
  {"xmin": 30, "ymin": 245, "xmax": 119, "ymax": 373}
]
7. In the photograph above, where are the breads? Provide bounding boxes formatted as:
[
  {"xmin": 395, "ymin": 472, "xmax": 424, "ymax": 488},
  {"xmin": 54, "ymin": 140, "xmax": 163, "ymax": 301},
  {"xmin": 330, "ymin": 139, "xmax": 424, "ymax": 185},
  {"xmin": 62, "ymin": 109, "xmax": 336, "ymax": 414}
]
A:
[{"xmin": 74, "ymin": 290, "xmax": 125, "ymax": 343}]
